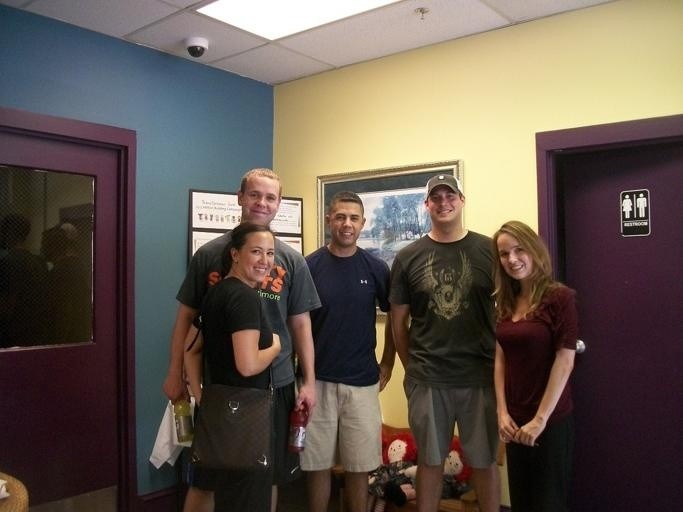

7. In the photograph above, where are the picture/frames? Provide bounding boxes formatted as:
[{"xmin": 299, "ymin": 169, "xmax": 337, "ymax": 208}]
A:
[
  {"xmin": 185, "ymin": 187, "xmax": 304, "ymax": 279},
  {"xmin": 313, "ymin": 157, "xmax": 465, "ymax": 322}
]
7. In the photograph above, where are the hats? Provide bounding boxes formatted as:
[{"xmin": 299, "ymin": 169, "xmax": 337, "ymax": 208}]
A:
[{"xmin": 426, "ymin": 174, "xmax": 462, "ymax": 200}]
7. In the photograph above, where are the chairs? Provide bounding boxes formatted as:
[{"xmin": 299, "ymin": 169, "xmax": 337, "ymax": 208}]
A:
[{"xmin": 327, "ymin": 416, "xmax": 505, "ymax": 512}]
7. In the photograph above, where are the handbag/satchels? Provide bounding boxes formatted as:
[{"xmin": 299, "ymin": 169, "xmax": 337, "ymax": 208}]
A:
[{"xmin": 191, "ymin": 383, "xmax": 274, "ymax": 472}]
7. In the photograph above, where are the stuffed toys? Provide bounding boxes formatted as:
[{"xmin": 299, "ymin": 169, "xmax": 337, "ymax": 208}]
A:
[
  {"xmin": 387, "ymin": 439, "xmax": 471, "ymax": 507},
  {"xmin": 337, "ymin": 434, "xmax": 417, "ymax": 498}
]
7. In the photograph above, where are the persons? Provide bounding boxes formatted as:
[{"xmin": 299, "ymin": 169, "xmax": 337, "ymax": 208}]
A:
[
  {"xmin": 0, "ymin": 212, "xmax": 92, "ymax": 348},
  {"xmin": 388, "ymin": 174, "xmax": 502, "ymax": 512},
  {"xmin": 491, "ymin": 221, "xmax": 578, "ymax": 512},
  {"xmin": 163, "ymin": 169, "xmax": 317, "ymax": 512},
  {"xmin": 295, "ymin": 191, "xmax": 395, "ymax": 512},
  {"xmin": 184, "ymin": 220, "xmax": 281, "ymax": 512}
]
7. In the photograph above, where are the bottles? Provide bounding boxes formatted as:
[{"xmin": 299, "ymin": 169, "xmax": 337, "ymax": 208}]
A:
[
  {"xmin": 173, "ymin": 395, "xmax": 193, "ymax": 442},
  {"xmin": 287, "ymin": 403, "xmax": 306, "ymax": 451}
]
